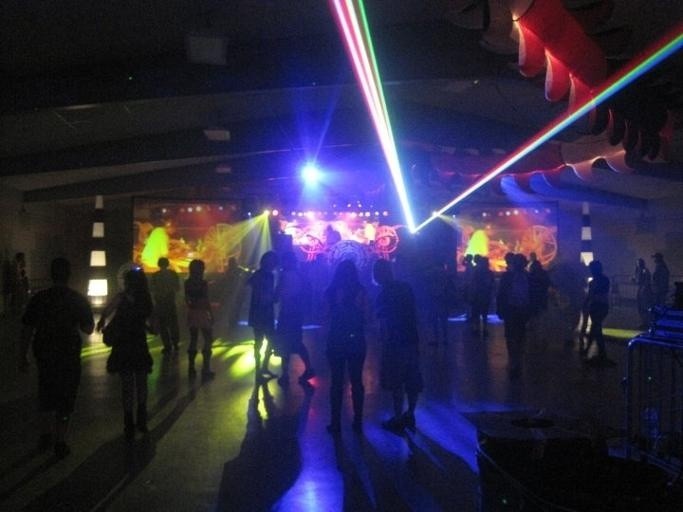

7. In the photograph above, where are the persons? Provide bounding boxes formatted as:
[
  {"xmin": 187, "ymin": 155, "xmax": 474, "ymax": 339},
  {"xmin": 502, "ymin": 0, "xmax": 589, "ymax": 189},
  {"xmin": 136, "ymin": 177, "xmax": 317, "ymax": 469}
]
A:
[
  {"xmin": 222, "ymin": 255, "xmax": 250, "ymax": 328},
  {"xmin": 3, "ymin": 252, "xmax": 30, "ymax": 322},
  {"xmin": 151, "ymin": 257, "xmax": 181, "ymax": 355},
  {"xmin": 184, "ymin": 259, "xmax": 216, "ymax": 377},
  {"xmin": 97, "ymin": 266, "xmax": 160, "ymax": 444},
  {"xmin": 651, "ymin": 252, "xmax": 670, "ymax": 322},
  {"xmin": 427, "ymin": 262, "xmax": 458, "ymax": 346},
  {"xmin": 496, "ymin": 252, "xmax": 551, "ymax": 381},
  {"xmin": 322, "ymin": 258, "xmax": 366, "ymax": 434},
  {"xmin": 462, "ymin": 255, "xmax": 495, "ymax": 323},
  {"xmin": 244, "ymin": 251, "xmax": 280, "ymax": 370},
  {"xmin": 370, "ymin": 258, "xmax": 422, "ymax": 435},
  {"xmin": 554, "ymin": 261, "xmax": 609, "ymax": 354},
  {"xmin": 19, "ymin": 258, "xmax": 94, "ymax": 455},
  {"xmin": 273, "ymin": 252, "xmax": 316, "ymax": 383},
  {"xmin": 633, "ymin": 256, "xmax": 652, "ymax": 329}
]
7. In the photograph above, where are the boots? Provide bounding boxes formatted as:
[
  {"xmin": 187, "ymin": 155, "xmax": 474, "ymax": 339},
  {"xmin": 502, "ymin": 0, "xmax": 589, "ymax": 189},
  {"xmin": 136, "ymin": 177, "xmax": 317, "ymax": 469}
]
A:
[
  {"xmin": 201, "ymin": 350, "xmax": 215, "ymax": 376},
  {"xmin": 187, "ymin": 349, "xmax": 196, "ymax": 377}
]
